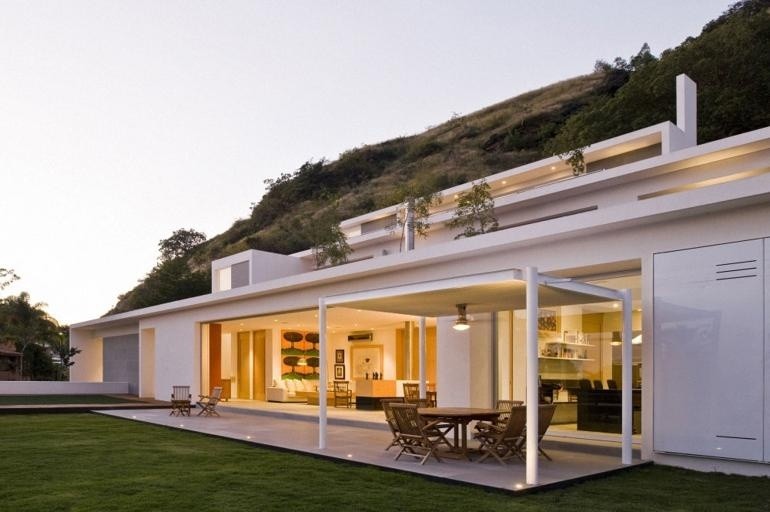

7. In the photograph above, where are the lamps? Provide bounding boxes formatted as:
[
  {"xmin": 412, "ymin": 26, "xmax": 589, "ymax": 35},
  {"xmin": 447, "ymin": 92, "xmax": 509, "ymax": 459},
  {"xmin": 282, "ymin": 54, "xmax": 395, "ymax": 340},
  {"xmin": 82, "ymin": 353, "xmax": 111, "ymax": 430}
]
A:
[
  {"xmin": 452, "ymin": 303, "xmax": 470, "ymax": 331},
  {"xmin": 610, "ymin": 331, "xmax": 622, "ymax": 347}
]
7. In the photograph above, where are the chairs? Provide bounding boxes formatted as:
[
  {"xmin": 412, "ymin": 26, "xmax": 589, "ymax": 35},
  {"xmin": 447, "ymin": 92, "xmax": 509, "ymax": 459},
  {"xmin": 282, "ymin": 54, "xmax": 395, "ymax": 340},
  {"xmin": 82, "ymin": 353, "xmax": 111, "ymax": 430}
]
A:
[
  {"xmin": 379, "ymin": 383, "xmax": 557, "ymax": 466},
  {"xmin": 579, "ymin": 378, "xmax": 618, "ymax": 390},
  {"xmin": 170, "ymin": 385, "xmax": 192, "ymax": 416},
  {"xmin": 195, "ymin": 386, "xmax": 224, "ymax": 417},
  {"xmin": 333, "ymin": 380, "xmax": 353, "ymax": 409}
]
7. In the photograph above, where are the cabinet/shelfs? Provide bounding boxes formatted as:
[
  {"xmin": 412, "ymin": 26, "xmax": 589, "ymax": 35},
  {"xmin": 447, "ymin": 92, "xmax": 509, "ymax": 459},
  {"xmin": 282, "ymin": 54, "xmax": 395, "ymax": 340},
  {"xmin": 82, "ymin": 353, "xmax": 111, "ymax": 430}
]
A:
[
  {"xmin": 220, "ymin": 378, "xmax": 231, "ymax": 401},
  {"xmin": 539, "ymin": 341, "xmax": 597, "ymax": 362}
]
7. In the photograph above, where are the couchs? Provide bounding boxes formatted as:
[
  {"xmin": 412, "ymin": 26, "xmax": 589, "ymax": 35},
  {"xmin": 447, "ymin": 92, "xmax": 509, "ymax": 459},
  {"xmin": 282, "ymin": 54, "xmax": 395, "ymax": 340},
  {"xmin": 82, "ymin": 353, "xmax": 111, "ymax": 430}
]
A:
[{"xmin": 266, "ymin": 386, "xmax": 319, "ymax": 403}]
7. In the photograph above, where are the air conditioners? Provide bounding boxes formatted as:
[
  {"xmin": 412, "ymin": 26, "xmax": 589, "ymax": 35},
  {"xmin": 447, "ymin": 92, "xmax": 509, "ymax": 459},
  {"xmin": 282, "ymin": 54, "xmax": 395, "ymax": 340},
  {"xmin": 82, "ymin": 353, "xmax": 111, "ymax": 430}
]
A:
[{"xmin": 346, "ymin": 331, "xmax": 374, "ymax": 345}]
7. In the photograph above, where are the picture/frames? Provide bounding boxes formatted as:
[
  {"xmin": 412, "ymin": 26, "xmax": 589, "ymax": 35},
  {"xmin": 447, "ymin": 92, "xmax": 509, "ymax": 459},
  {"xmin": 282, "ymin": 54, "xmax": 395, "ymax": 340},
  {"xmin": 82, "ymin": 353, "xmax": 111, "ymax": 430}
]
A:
[
  {"xmin": 335, "ymin": 349, "xmax": 344, "ymax": 363},
  {"xmin": 537, "ymin": 306, "xmax": 561, "ymax": 338},
  {"xmin": 350, "ymin": 344, "xmax": 385, "ymax": 382},
  {"xmin": 334, "ymin": 364, "xmax": 346, "ymax": 380}
]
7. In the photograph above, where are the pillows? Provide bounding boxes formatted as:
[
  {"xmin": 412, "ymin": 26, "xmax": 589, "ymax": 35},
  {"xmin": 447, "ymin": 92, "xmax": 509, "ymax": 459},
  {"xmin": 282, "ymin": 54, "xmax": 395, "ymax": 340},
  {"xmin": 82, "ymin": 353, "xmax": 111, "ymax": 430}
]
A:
[{"xmin": 273, "ymin": 378, "xmax": 313, "ymax": 392}]
